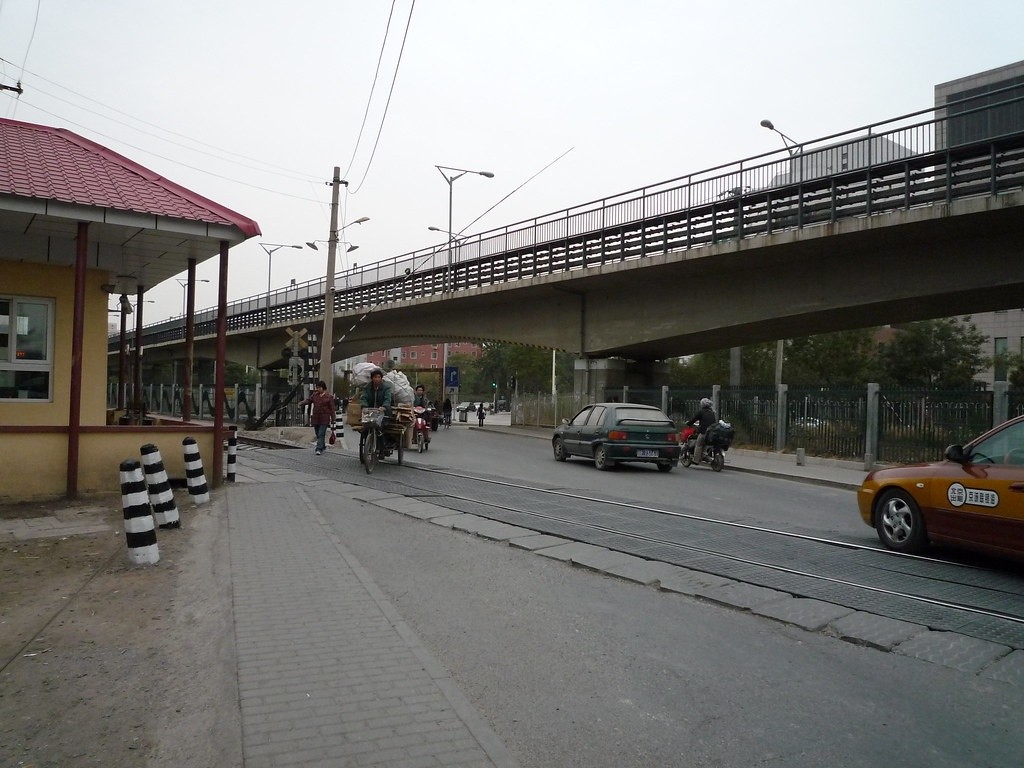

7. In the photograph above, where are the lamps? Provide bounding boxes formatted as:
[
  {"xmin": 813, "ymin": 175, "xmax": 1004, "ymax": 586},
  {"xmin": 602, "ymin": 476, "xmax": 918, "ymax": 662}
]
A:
[{"xmin": 119, "ymin": 295, "xmax": 132, "ymax": 315}]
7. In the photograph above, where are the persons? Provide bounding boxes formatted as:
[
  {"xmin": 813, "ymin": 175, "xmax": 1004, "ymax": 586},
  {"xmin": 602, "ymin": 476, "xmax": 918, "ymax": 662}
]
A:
[
  {"xmin": 686, "ymin": 398, "xmax": 716, "ymax": 465},
  {"xmin": 359, "ymin": 370, "xmax": 392, "ymax": 460},
  {"xmin": 414, "ymin": 384, "xmax": 435, "ymax": 426},
  {"xmin": 297, "ymin": 381, "xmax": 336, "ymax": 455},
  {"xmin": 477, "ymin": 403, "xmax": 486, "ymax": 427},
  {"xmin": 488, "ymin": 400, "xmax": 511, "ymax": 415},
  {"xmin": 443, "ymin": 398, "xmax": 453, "ymax": 426}
]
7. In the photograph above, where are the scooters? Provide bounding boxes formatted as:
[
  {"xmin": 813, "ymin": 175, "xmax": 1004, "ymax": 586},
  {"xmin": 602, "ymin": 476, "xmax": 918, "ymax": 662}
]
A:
[{"xmin": 413, "ymin": 406, "xmax": 432, "ymax": 453}]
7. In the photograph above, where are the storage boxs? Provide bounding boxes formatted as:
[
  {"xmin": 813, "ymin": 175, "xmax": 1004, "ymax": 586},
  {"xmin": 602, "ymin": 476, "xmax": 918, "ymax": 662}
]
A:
[
  {"xmin": 403, "ymin": 428, "xmax": 413, "ymax": 449},
  {"xmin": 345, "ymin": 403, "xmax": 362, "ymax": 425}
]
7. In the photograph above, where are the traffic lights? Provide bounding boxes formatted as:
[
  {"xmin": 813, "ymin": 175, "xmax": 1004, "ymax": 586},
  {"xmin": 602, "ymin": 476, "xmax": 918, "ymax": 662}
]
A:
[
  {"xmin": 511, "ymin": 376, "xmax": 514, "ymax": 388},
  {"xmin": 493, "ymin": 382, "xmax": 496, "ymax": 387}
]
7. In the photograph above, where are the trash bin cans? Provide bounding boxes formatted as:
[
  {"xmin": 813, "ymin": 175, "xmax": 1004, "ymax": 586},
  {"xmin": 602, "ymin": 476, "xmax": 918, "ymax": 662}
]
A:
[{"xmin": 458, "ymin": 408, "xmax": 468, "ymax": 422}]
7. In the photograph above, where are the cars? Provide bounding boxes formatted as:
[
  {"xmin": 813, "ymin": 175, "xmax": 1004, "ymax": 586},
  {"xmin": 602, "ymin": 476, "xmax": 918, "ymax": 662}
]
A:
[
  {"xmin": 552, "ymin": 403, "xmax": 681, "ymax": 472},
  {"xmin": 425, "ymin": 398, "xmax": 439, "ymax": 431},
  {"xmin": 456, "ymin": 402, "xmax": 476, "ymax": 412},
  {"xmin": 794, "ymin": 417, "xmax": 824, "ymax": 428},
  {"xmin": 857, "ymin": 415, "xmax": 1024, "ymax": 563},
  {"xmin": 474, "ymin": 402, "xmax": 481, "ymax": 409},
  {"xmin": 490, "ymin": 400, "xmax": 506, "ymax": 412},
  {"xmin": 483, "ymin": 403, "xmax": 492, "ymax": 411}
]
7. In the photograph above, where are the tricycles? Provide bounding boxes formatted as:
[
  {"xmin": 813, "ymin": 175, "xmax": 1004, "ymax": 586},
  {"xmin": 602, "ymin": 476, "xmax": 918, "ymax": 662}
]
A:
[{"xmin": 358, "ymin": 408, "xmax": 406, "ymax": 474}]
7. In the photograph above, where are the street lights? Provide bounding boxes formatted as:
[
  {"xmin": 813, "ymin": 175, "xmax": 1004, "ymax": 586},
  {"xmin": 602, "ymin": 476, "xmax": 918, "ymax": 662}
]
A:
[
  {"xmin": 306, "ymin": 217, "xmax": 371, "ymax": 396},
  {"xmin": 429, "ymin": 227, "xmax": 470, "ymax": 291},
  {"xmin": 435, "ymin": 165, "xmax": 495, "ymax": 293},
  {"xmin": 130, "ymin": 279, "xmax": 210, "ymax": 329},
  {"xmin": 761, "ymin": 120, "xmax": 800, "ymax": 388},
  {"xmin": 259, "ymin": 243, "xmax": 303, "ymax": 292}
]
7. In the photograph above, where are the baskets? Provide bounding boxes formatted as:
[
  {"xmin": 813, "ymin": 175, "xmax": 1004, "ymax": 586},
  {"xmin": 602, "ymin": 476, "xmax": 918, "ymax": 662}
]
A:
[{"xmin": 361, "ymin": 407, "xmax": 385, "ymax": 427}]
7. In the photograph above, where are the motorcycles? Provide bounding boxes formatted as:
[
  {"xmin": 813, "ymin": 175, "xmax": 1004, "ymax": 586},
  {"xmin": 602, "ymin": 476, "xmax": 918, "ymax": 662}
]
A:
[{"xmin": 679, "ymin": 421, "xmax": 725, "ymax": 472}]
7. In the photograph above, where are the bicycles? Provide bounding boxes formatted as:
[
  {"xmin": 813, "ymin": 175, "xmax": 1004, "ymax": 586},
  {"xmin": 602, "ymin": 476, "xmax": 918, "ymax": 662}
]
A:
[{"xmin": 444, "ymin": 412, "xmax": 451, "ymax": 430}]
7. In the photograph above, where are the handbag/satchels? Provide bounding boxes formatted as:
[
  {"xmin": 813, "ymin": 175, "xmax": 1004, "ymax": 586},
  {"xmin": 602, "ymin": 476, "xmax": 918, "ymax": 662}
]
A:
[{"xmin": 328, "ymin": 432, "xmax": 337, "ymax": 445}]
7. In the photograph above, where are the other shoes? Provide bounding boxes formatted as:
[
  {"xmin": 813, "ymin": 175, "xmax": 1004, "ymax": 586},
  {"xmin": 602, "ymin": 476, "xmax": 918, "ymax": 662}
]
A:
[
  {"xmin": 689, "ymin": 458, "xmax": 699, "ymax": 465},
  {"xmin": 316, "ymin": 451, "xmax": 321, "ymax": 455}
]
